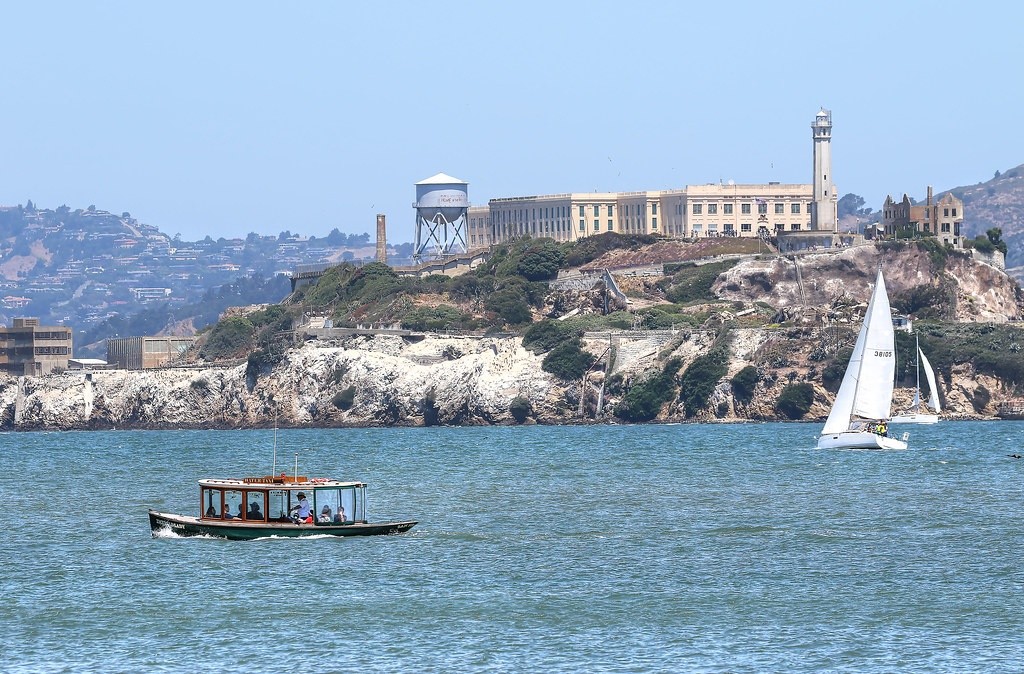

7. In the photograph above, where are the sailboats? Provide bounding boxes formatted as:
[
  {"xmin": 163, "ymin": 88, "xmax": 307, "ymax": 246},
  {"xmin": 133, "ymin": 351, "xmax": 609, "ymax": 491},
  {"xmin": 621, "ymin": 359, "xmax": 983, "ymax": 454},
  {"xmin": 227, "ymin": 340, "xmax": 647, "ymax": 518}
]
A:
[
  {"xmin": 888, "ymin": 330, "xmax": 943, "ymax": 424},
  {"xmin": 817, "ymin": 260, "xmax": 911, "ymax": 450}
]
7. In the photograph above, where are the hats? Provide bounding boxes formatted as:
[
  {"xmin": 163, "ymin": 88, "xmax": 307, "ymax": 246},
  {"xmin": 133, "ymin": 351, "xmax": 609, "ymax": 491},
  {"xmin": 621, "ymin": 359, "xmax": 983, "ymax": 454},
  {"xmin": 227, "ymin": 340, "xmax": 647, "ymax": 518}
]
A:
[
  {"xmin": 296, "ymin": 492, "xmax": 306, "ymax": 499},
  {"xmin": 250, "ymin": 502, "xmax": 260, "ymax": 510}
]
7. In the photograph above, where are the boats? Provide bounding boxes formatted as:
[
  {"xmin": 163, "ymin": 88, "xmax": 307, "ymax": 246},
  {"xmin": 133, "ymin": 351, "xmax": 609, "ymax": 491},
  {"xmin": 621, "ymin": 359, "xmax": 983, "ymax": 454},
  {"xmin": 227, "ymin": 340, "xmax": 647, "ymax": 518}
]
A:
[{"xmin": 147, "ymin": 403, "xmax": 419, "ymax": 541}]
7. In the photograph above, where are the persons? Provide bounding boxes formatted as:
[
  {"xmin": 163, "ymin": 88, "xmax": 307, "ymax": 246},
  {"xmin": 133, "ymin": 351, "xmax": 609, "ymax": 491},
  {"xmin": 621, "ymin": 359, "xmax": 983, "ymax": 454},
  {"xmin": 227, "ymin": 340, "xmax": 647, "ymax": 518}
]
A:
[
  {"xmin": 334, "ymin": 507, "xmax": 347, "ymax": 522},
  {"xmin": 877, "ymin": 422, "xmax": 888, "ymax": 437},
  {"xmin": 735, "ymin": 230, "xmax": 737, "ymax": 237},
  {"xmin": 299, "ymin": 510, "xmax": 313, "ymax": 524},
  {"xmin": 758, "ymin": 228, "xmax": 764, "ymax": 239},
  {"xmin": 206, "ymin": 502, "xmax": 263, "ymax": 520},
  {"xmin": 288, "ymin": 492, "xmax": 310, "ymax": 520},
  {"xmin": 705, "ymin": 230, "xmax": 718, "ymax": 236},
  {"xmin": 691, "ymin": 229, "xmax": 698, "ymax": 237},
  {"xmin": 683, "ymin": 229, "xmax": 685, "ymax": 237},
  {"xmin": 773, "ymin": 227, "xmax": 784, "ymax": 236},
  {"xmin": 319, "ymin": 505, "xmax": 334, "ymax": 522}
]
